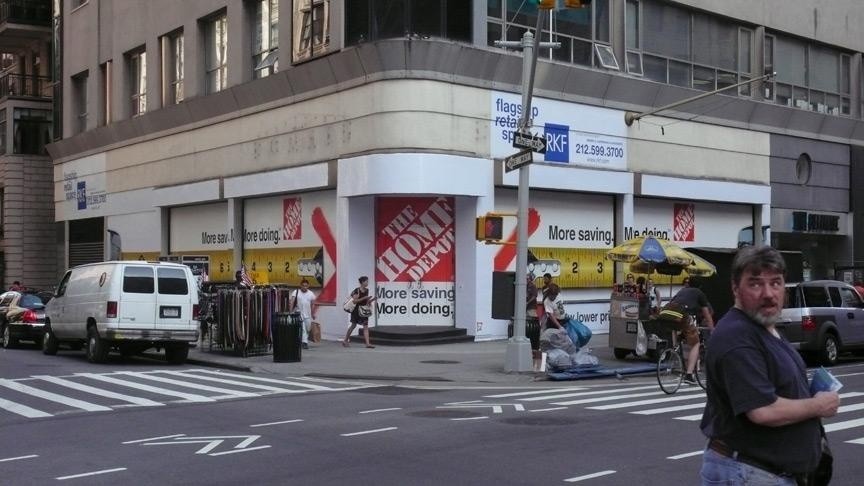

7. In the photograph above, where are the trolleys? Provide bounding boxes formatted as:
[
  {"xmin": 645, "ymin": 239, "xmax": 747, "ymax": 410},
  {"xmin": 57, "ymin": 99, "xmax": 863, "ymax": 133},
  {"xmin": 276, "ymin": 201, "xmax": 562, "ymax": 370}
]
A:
[{"xmin": 606, "ymin": 293, "xmax": 670, "ymax": 363}]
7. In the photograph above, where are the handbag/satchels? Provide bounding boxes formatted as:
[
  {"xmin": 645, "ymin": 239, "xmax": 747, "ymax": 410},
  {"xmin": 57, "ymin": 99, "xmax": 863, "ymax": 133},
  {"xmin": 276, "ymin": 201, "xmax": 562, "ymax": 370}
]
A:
[
  {"xmin": 307, "ymin": 314, "xmax": 322, "ymax": 343},
  {"xmin": 797, "ymin": 433, "xmax": 834, "ymax": 486},
  {"xmin": 359, "ymin": 305, "xmax": 372, "ymax": 318},
  {"xmin": 288, "ymin": 288, "xmax": 298, "ymax": 309},
  {"xmin": 343, "ymin": 288, "xmax": 360, "ymax": 313},
  {"xmin": 657, "ymin": 303, "xmax": 686, "ymax": 327}
]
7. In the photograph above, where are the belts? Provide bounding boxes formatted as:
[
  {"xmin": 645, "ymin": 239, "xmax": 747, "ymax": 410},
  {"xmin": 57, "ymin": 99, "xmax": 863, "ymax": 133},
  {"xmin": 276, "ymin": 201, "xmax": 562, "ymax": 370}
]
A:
[{"xmin": 709, "ymin": 438, "xmax": 795, "ymax": 480}]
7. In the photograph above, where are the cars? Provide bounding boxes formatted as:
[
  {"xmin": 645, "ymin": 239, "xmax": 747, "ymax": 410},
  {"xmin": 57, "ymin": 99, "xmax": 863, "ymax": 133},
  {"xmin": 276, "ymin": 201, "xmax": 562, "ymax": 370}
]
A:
[{"xmin": 0, "ymin": 290, "xmax": 58, "ymax": 349}]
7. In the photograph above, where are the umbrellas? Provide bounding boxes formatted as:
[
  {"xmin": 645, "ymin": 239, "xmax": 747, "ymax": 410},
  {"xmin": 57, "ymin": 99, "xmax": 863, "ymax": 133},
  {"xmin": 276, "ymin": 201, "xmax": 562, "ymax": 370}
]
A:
[
  {"xmin": 607, "ymin": 234, "xmax": 696, "ymax": 296},
  {"xmin": 630, "ymin": 249, "xmax": 717, "ymax": 301}
]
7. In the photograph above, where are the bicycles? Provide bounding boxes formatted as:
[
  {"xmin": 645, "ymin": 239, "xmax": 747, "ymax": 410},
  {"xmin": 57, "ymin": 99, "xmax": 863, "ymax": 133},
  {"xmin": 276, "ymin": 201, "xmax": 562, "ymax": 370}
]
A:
[{"xmin": 655, "ymin": 324, "xmax": 712, "ymax": 396}]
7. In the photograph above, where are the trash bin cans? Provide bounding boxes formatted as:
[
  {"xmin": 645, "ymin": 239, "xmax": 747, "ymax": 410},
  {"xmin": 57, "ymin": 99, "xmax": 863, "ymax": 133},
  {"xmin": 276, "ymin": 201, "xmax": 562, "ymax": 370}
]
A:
[
  {"xmin": 508, "ymin": 315, "xmax": 541, "ymax": 351},
  {"xmin": 271, "ymin": 311, "xmax": 303, "ymax": 363}
]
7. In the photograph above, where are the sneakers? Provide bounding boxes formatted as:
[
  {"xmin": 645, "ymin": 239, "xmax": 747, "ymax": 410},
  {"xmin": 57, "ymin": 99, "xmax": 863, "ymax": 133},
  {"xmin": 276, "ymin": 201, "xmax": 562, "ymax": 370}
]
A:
[
  {"xmin": 365, "ymin": 343, "xmax": 376, "ymax": 348},
  {"xmin": 343, "ymin": 341, "xmax": 352, "ymax": 347},
  {"xmin": 302, "ymin": 342, "xmax": 309, "ymax": 350},
  {"xmin": 684, "ymin": 373, "xmax": 697, "ymax": 384}
]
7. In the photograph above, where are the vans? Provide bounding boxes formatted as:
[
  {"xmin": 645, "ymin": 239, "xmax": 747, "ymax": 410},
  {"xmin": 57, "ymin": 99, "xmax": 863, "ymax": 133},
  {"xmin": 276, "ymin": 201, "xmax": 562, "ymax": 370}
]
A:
[{"xmin": 771, "ymin": 279, "xmax": 863, "ymax": 365}]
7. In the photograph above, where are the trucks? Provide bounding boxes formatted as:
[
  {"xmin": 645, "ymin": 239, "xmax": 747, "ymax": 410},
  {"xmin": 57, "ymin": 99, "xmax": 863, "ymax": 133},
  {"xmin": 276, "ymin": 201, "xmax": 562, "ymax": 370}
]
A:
[{"xmin": 42, "ymin": 261, "xmax": 202, "ymax": 365}]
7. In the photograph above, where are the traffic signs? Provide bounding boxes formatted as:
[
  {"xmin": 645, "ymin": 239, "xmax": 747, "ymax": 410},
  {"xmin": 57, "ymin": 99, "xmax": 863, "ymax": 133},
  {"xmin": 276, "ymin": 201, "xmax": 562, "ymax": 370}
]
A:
[
  {"xmin": 504, "ymin": 147, "xmax": 533, "ymax": 172},
  {"xmin": 512, "ymin": 131, "xmax": 547, "ymax": 155}
]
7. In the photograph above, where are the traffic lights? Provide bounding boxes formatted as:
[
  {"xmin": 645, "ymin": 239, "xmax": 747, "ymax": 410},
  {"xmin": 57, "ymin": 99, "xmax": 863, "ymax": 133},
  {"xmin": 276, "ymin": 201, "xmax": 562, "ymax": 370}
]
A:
[{"xmin": 475, "ymin": 216, "xmax": 504, "ymax": 243}]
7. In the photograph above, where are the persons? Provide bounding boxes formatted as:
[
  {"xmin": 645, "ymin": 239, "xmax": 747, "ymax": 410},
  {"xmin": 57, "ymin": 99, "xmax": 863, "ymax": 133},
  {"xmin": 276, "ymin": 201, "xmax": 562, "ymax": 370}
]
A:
[
  {"xmin": 9, "ymin": 280, "xmax": 24, "ymax": 292},
  {"xmin": 343, "ymin": 277, "xmax": 377, "ymax": 348},
  {"xmin": 659, "ymin": 274, "xmax": 715, "ymax": 385},
  {"xmin": 236, "ymin": 271, "xmax": 247, "ymax": 288},
  {"xmin": 682, "ymin": 277, "xmax": 690, "ymax": 289},
  {"xmin": 289, "ymin": 280, "xmax": 316, "ymax": 349},
  {"xmin": 542, "ymin": 273, "xmax": 562, "ymax": 310},
  {"xmin": 699, "ymin": 244, "xmax": 840, "ymax": 485},
  {"xmin": 624, "ymin": 272, "xmax": 661, "ymax": 313},
  {"xmin": 527, "ymin": 272, "xmax": 538, "ymax": 310}
]
